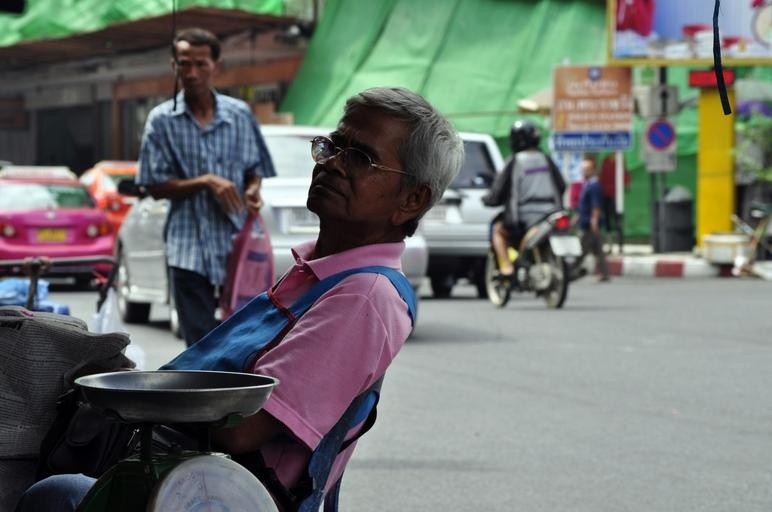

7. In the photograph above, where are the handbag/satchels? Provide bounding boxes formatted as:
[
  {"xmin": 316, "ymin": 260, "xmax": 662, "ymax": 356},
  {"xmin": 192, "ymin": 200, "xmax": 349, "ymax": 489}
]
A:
[{"xmin": 220, "ymin": 209, "xmax": 274, "ymax": 320}]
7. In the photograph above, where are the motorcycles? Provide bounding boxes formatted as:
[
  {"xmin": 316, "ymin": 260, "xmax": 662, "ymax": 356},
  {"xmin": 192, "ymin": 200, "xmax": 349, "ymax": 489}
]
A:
[{"xmin": 469, "ymin": 172, "xmax": 583, "ymax": 314}]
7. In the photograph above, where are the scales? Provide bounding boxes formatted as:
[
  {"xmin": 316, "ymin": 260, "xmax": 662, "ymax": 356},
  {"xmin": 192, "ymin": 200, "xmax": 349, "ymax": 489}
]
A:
[{"xmin": 73, "ymin": 369, "xmax": 281, "ymax": 512}]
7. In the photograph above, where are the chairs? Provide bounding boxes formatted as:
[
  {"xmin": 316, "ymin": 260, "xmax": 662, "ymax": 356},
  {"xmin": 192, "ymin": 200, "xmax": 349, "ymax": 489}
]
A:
[{"xmin": 72, "ymin": 372, "xmax": 381, "ymax": 512}]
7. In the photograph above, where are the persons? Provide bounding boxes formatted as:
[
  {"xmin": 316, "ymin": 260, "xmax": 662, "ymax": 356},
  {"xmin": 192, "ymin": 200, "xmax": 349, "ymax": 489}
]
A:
[
  {"xmin": 482, "ymin": 119, "xmax": 566, "ymax": 281},
  {"xmin": 14, "ymin": 87, "xmax": 465, "ymax": 512},
  {"xmin": 134, "ymin": 27, "xmax": 276, "ymax": 348},
  {"xmin": 577, "ymin": 150, "xmax": 629, "ymax": 282}
]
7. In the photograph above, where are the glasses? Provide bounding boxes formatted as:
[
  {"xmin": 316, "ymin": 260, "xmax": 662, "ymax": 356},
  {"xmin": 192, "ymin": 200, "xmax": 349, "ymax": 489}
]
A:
[{"xmin": 311, "ymin": 136, "xmax": 410, "ymax": 179}]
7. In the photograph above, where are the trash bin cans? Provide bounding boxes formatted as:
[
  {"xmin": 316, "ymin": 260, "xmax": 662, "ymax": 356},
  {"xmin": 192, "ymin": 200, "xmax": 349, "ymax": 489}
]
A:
[{"xmin": 658, "ymin": 183, "xmax": 693, "ymax": 250}]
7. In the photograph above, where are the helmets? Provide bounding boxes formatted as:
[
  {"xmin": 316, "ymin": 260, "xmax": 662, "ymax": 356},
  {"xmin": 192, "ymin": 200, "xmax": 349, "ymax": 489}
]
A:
[{"xmin": 512, "ymin": 119, "xmax": 540, "ymax": 145}]
